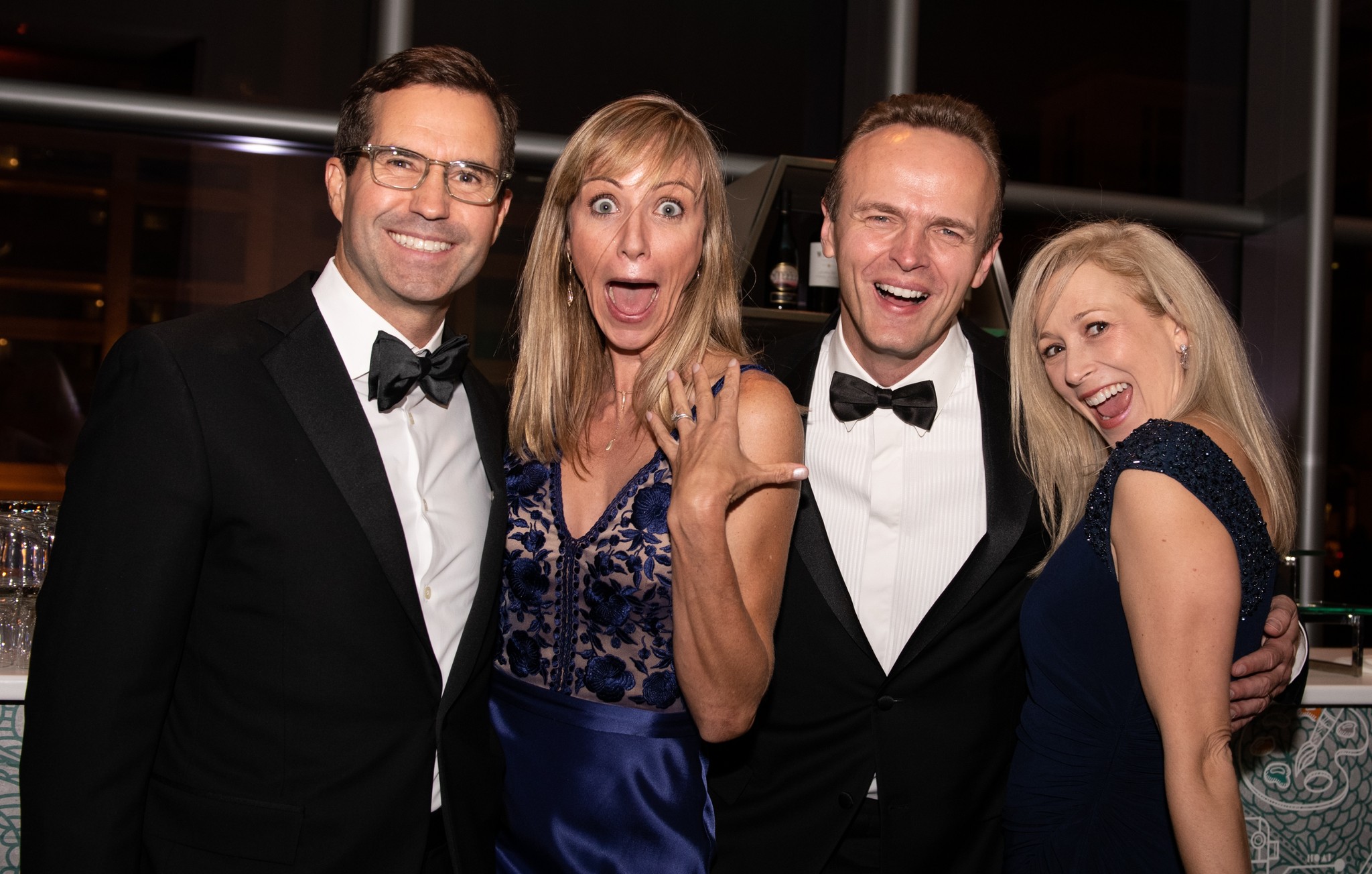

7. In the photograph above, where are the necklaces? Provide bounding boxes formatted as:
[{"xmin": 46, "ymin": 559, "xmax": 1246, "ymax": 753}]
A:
[{"xmin": 604, "ymin": 355, "xmax": 644, "ymax": 451}]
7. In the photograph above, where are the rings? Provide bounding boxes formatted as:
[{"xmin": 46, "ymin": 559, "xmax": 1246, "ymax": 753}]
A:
[{"xmin": 670, "ymin": 412, "xmax": 695, "ymax": 428}]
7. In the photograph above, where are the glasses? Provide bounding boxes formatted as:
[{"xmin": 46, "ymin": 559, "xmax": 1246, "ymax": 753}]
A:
[{"xmin": 340, "ymin": 144, "xmax": 512, "ymax": 205}]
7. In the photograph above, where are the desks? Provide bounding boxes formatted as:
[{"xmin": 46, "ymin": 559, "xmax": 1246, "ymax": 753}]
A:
[{"xmin": 1229, "ymin": 648, "xmax": 1372, "ymax": 874}]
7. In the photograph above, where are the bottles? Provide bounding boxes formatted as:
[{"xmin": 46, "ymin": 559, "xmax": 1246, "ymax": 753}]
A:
[
  {"xmin": 765, "ymin": 197, "xmax": 802, "ymax": 310},
  {"xmin": 805, "ymin": 214, "xmax": 839, "ymax": 313}
]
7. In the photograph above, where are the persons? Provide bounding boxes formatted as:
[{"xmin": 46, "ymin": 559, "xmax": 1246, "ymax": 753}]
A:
[
  {"xmin": 710, "ymin": 92, "xmax": 1309, "ymax": 874},
  {"xmin": 476, "ymin": 95, "xmax": 809, "ymax": 874},
  {"xmin": 18, "ymin": 44, "xmax": 514, "ymax": 874}
]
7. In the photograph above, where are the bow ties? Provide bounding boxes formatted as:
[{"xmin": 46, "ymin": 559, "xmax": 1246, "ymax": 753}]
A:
[
  {"xmin": 829, "ymin": 371, "xmax": 937, "ymax": 431},
  {"xmin": 368, "ymin": 330, "xmax": 469, "ymax": 411}
]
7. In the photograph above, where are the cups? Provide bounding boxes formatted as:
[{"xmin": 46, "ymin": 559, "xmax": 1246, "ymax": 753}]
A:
[{"xmin": 0, "ymin": 500, "xmax": 54, "ymax": 600}]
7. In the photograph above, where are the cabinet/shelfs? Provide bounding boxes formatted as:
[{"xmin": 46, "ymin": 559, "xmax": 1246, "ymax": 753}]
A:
[{"xmin": 723, "ymin": 154, "xmax": 1014, "ymax": 343}]
